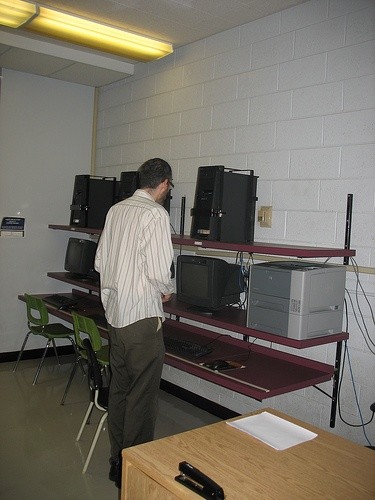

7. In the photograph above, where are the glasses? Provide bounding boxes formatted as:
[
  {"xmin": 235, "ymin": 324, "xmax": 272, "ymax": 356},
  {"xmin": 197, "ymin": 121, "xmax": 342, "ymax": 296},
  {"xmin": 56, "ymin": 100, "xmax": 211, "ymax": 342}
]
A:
[{"xmin": 162, "ymin": 179, "xmax": 174, "ymax": 190}]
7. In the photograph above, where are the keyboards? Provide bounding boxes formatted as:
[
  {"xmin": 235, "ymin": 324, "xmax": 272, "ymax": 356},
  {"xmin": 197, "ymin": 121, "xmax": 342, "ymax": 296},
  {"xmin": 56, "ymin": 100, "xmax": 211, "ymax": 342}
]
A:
[
  {"xmin": 164, "ymin": 336, "xmax": 213, "ymax": 359},
  {"xmin": 45, "ymin": 295, "xmax": 79, "ymax": 310}
]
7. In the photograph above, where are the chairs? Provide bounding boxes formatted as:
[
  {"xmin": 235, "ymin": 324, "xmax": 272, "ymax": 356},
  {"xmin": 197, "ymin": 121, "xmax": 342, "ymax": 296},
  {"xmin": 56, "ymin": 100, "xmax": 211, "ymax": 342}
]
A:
[{"xmin": 13, "ymin": 293, "xmax": 112, "ymax": 477}]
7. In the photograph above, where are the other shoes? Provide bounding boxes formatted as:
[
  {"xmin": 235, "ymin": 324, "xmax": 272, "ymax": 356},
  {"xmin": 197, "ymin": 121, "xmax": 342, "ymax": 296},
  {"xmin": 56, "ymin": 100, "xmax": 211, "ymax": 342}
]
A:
[{"xmin": 109, "ymin": 468, "xmax": 121, "ymax": 488}]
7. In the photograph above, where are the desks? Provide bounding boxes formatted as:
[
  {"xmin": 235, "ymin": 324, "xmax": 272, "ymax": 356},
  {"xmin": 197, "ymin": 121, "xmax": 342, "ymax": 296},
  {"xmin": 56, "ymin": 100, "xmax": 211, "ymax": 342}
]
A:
[{"xmin": 121, "ymin": 408, "xmax": 375, "ymax": 500}]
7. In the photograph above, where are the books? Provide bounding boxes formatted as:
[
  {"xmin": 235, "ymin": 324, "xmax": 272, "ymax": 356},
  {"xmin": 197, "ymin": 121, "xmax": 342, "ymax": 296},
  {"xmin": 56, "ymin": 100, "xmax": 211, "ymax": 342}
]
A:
[{"xmin": 227, "ymin": 412, "xmax": 319, "ymax": 451}]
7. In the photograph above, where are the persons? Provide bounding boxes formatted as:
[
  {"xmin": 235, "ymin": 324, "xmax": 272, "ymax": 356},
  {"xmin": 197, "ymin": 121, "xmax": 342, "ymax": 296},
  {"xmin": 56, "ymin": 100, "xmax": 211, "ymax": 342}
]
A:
[{"xmin": 95, "ymin": 157, "xmax": 174, "ymax": 488}]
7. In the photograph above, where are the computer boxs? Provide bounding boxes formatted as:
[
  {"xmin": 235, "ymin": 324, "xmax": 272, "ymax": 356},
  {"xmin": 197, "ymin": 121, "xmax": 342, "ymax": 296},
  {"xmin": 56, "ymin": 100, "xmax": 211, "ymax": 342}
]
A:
[
  {"xmin": 120, "ymin": 172, "xmax": 171, "ymax": 218},
  {"xmin": 190, "ymin": 166, "xmax": 257, "ymax": 242},
  {"xmin": 70, "ymin": 175, "xmax": 115, "ymax": 230}
]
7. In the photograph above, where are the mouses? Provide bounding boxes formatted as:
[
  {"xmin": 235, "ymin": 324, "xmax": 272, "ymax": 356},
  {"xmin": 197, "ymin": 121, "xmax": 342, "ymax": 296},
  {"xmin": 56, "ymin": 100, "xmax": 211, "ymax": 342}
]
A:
[
  {"xmin": 210, "ymin": 359, "xmax": 229, "ymax": 370},
  {"xmin": 59, "ymin": 305, "xmax": 76, "ymax": 310}
]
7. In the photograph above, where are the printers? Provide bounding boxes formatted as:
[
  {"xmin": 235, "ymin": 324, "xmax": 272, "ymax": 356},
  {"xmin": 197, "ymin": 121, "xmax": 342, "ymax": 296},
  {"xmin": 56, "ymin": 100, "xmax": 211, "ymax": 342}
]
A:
[{"xmin": 246, "ymin": 252, "xmax": 345, "ymax": 342}]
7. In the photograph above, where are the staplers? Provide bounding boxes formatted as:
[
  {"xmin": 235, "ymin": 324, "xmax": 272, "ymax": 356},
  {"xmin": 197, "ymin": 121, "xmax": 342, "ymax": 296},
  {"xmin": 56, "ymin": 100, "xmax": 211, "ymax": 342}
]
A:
[{"xmin": 174, "ymin": 461, "xmax": 224, "ymax": 500}]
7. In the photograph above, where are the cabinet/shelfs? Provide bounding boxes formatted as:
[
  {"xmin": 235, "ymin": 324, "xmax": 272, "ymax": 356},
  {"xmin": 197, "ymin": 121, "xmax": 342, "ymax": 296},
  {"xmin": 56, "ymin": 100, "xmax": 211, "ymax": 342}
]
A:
[{"xmin": 46, "ymin": 184, "xmax": 355, "ymax": 428}]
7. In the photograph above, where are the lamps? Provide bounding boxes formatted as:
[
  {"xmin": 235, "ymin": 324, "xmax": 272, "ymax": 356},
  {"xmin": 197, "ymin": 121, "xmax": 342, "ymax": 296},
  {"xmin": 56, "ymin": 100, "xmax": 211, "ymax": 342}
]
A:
[{"xmin": 0, "ymin": 0, "xmax": 175, "ymax": 62}]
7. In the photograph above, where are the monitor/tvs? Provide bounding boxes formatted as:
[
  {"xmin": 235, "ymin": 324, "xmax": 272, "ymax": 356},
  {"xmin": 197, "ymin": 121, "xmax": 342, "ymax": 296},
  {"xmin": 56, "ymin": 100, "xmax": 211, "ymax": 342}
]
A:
[
  {"xmin": 65, "ymin": 237, "xmax": 97, "ymax": 281},
  {"xmin": 178, "ymin": 255, "xmax": 244, "ymax": 312}
]
